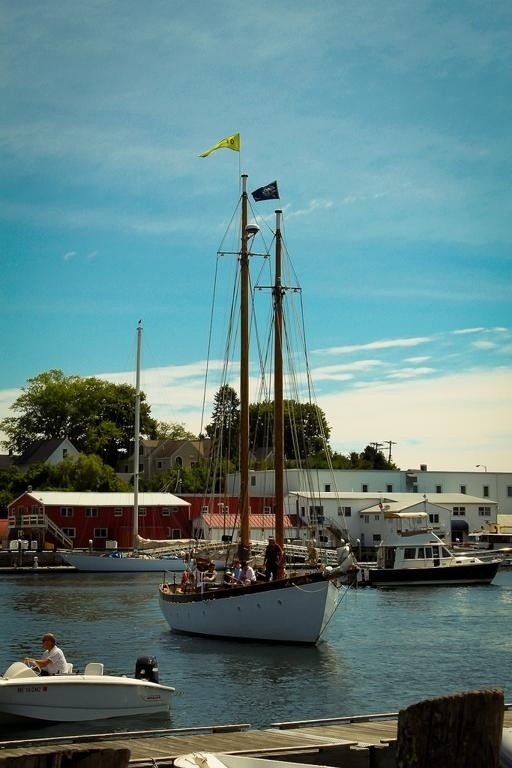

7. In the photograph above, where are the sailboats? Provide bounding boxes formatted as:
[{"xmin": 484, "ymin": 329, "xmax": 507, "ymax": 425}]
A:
[
  {"xmin": 58, "ymin": 318, "xmax": 229, "ymax": 574},
  {"xmin": 160, "ymin": 129, "xmax": 361, "ymax": 646}
]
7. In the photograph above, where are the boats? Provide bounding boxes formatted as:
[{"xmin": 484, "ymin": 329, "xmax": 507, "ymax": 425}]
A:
[
  {"xmin": 172, "ymin": 748, "xmax": 342, "ymax": 767},
  {"xmin": 450, "ymin": 546, "xmax": 512, "ymax": 567},
  {"xmin": 356, "ymin": 509, "xmax": 502, "ymax": 588},
  {"xmin": 0, "ymin": 660, "xmax": 176, "ymax": 723}
]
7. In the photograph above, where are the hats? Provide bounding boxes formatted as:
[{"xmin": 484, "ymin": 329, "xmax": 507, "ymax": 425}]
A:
[
  {"xmin": 267, "ymin": 535, "xmax": 275, "ymax": 540},
  {"xmin": 209, "ymin": 563, "xmax": 216, "ymax": 568},
  {"xmin": 239, "ymin": 560, "xmax": 248, "ymax": 568}
]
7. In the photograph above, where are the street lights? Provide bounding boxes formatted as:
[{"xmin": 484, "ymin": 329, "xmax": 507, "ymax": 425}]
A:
[{"xmin": 474, "ymin": 463, "xmax": 487, "ymax": 471}]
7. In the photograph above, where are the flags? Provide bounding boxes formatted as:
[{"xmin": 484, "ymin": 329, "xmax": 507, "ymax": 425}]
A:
[
  {"xmin": 200, "ymin": 132, "xmax": 239, "ymax": 158},
  {"xmin": 252, "ymin": 181, "xmax": 280, "ymax": 201}
]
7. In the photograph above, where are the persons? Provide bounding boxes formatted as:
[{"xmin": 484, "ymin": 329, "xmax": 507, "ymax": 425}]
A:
[
  {"xmin": 182, "ymin": 537, "xmax": 283, "ymax": 592},
  {"xmin": 24, "ymin": 633, "xmax": 68, "ymax": 676}
]
